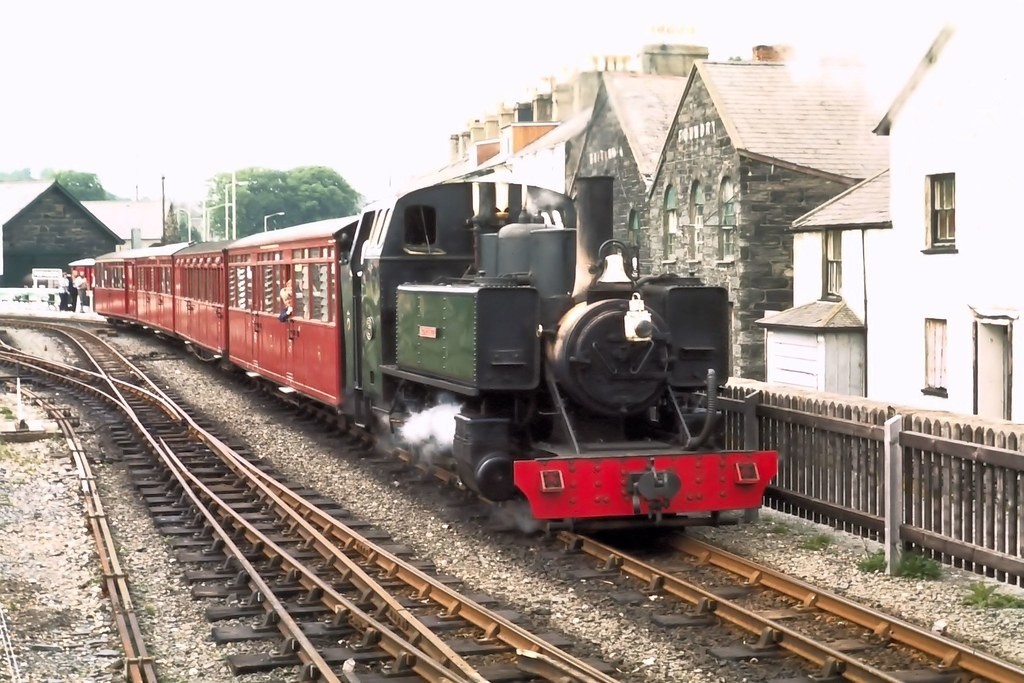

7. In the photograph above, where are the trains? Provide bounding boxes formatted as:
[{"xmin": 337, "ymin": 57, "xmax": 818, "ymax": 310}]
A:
[{"xmin": 68, "ymin": 174, "xmax": 777, "ymax": 523}]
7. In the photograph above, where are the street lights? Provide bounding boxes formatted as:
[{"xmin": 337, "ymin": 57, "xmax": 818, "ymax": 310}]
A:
[
  {"xmin": 223, "ymin": 182, "xmax": 253, "ymax": 237},
  {"xmin": 174, "ymin": 207, "xmax": 194, "ymax": 244},
  {"xmin": 206, "ymin": 200, "xmax": 233, "ymax": 241},
  {"xmin": 262, "ymin": 210, "xmax": 286, "ymax": 233}
]
